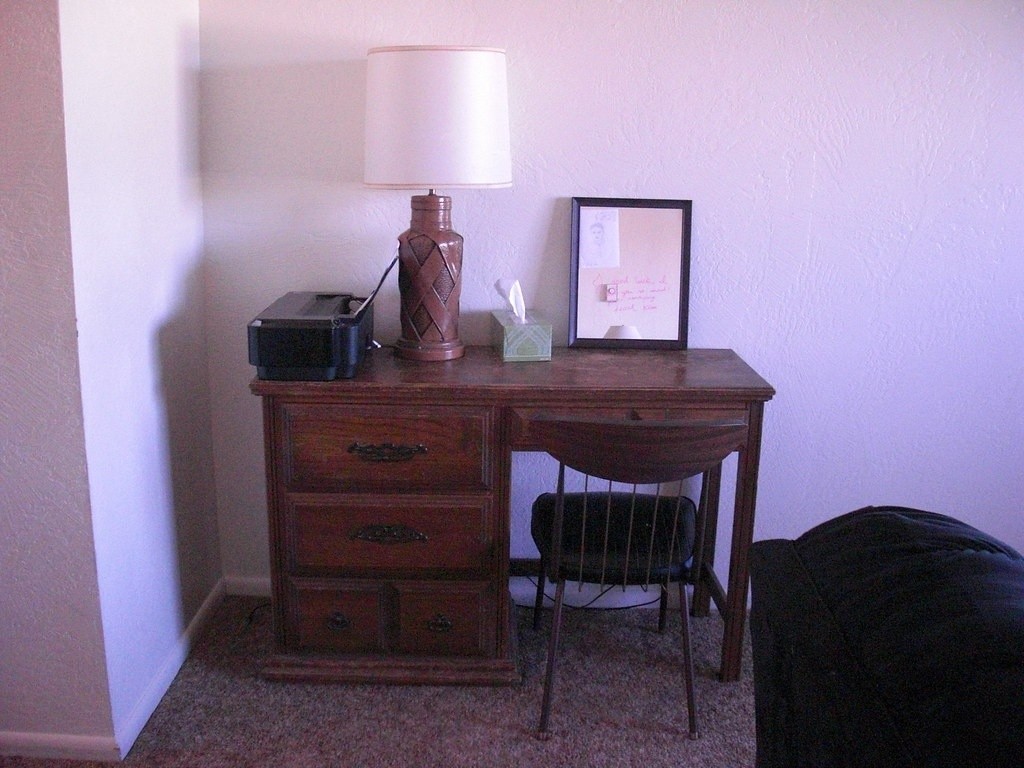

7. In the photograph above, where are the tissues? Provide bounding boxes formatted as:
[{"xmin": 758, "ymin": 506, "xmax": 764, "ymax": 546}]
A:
[{"xmin": 489, "ymin": 275, "xmax": 552, "ymax": 362}]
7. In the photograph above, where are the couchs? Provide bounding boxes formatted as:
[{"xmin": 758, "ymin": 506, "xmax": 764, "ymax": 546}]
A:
[{"xmin": 748, "ymin": 506, "xmax": 1024, "ymax": 768}]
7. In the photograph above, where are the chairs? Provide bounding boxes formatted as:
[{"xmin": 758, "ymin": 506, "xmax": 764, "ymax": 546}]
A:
[{"xmin": 531, "ymin": 413, "xmax": 749, "ymax": 739}]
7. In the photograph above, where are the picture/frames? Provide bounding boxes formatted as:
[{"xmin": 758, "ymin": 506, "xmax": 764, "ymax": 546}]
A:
[{"xmin": 568, "ymin": 197, "xmax": 692, "ymax": 350}]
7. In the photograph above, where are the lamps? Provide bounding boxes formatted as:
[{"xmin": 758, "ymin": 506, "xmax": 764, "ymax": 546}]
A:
[{"xmin": 362, "ymin": 45, "xmax": 512, "ymax": 361}]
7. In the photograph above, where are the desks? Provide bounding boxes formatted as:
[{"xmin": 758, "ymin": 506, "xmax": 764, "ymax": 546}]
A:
[{"xmin": 249, "ymin": 345, "xmax": 776, "ymax": 685}]
